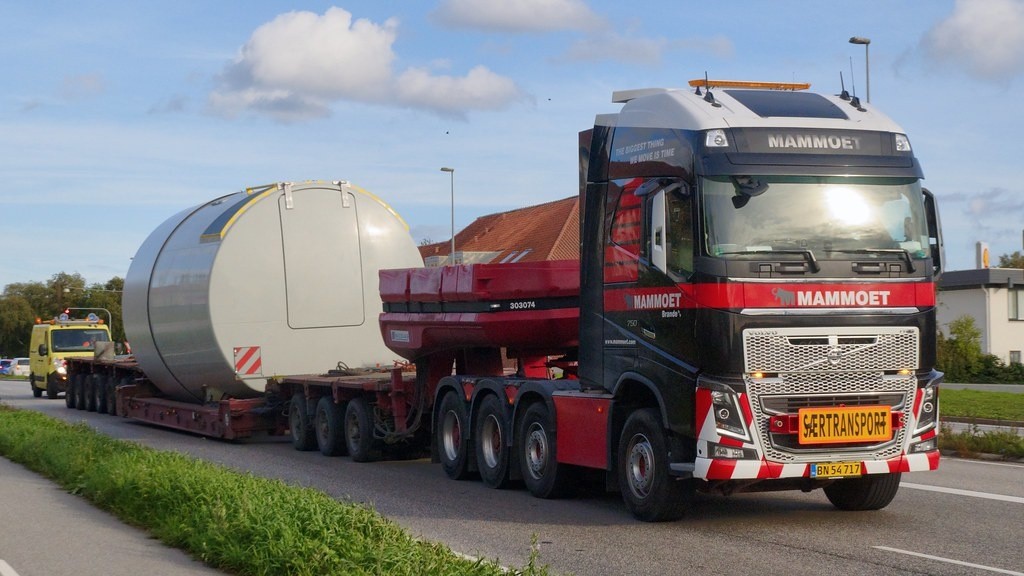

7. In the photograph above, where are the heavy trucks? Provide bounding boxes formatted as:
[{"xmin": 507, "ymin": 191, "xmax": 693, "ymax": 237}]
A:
[{"xmin": 61, "ymin": 72, "xmax": 946, "ymax": 531}]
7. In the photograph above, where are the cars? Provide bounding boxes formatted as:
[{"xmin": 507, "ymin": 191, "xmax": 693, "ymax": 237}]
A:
[{"xmin": 0, "ymin": 359, "xmax": 12, "ymax": 374}]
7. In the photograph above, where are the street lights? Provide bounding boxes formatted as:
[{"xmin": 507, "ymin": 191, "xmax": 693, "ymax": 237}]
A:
[
  {"xmin": 440, "ymin": 167, "xmax": 455, "ymax": 267},
  {"xmin": 849, "ymin": 37, "xmax": 871, "ymax": 104}
]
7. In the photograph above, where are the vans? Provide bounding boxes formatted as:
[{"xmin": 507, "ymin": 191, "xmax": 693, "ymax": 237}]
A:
[
  {"xmin": 28, "ymin": 317, "xmax": 117, "ymax": 400},
  {"xmin": 9, "ymin": 357, "xmax": 31, "ymax": 377}
]
